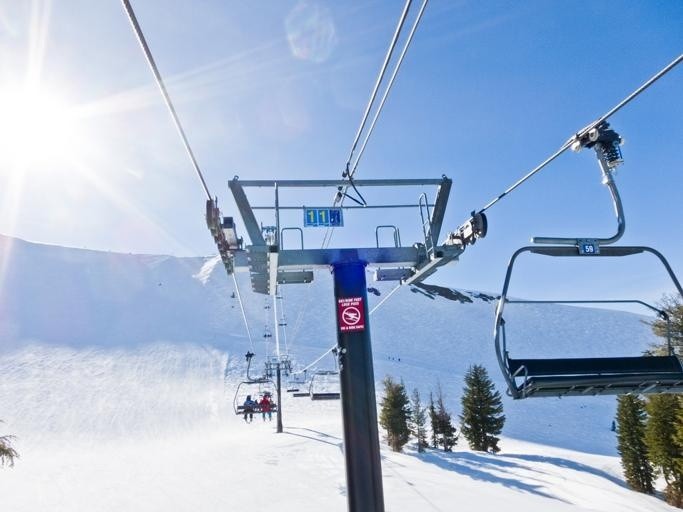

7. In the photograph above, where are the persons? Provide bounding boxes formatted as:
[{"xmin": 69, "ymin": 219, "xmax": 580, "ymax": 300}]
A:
[
  {"xmin": 258, "ymin": 395, "xmax": 272, "ymax": 421},
  {"xmin": 244, "ymin": 395, "xmax": 254, "ymax": 421}
]
